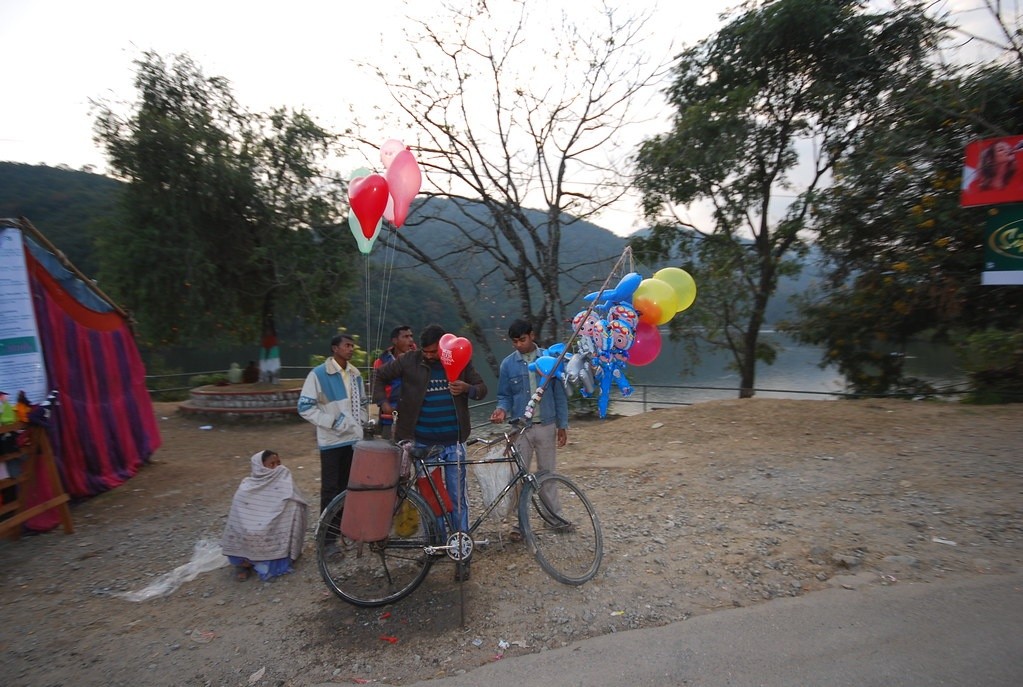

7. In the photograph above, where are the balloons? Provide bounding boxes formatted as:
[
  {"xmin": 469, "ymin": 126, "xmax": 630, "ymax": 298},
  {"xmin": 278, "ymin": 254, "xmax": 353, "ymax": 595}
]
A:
[
  {"xmin": 348, "ymin": 140, "xmax": 422, "ymax": 256},
  {"xmin": 528, "ymin": 267, "xmax": 696, "ymax": 420},
  {"xmin": 438, "ymin": 334, "xmax": 472, "ymax": 382}
]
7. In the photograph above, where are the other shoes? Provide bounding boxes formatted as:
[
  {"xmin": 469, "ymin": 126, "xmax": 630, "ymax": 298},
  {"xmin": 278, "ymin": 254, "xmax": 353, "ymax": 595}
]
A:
[
  {"xmin": 544, "ymin": 518, "xmax": 576, "ymax": 532},
  {"xmin": 416, "ymin": 548, "xmax": 448, "ymax": 567},
  {"xmin": 456, "ymin": 560, "xmax": 469, "ymax": 580},
  {"xmin": 509, "ymin": 522, "xmax": 522, "ymax": 542}
]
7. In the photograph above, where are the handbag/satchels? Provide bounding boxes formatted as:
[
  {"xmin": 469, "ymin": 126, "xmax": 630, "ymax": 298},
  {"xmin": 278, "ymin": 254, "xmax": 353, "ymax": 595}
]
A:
[{"xmin": 471, "ymin": 439, "xmax": 518, "ymax": 524}]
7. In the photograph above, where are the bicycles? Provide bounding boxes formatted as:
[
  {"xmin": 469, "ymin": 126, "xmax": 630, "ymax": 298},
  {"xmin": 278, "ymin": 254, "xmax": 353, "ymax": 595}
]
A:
[{"xmin": 313, "ymin": 414, "xmax": 604, "ymax": 607}]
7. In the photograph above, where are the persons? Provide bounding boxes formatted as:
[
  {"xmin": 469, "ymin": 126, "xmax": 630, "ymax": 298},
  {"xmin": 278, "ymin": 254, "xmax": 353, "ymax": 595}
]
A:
[
  {"xmin": 490, "ymin": 318, "xmax": 569, "ymax": 542},
  {"xmin": 372, "ymin": 325, "xmax": 487, "ymax": 582},
  {"xmin": 221, "ymin": 449, "xmax": 308, "ymax": 582},
  {"xmin": 977, "ymin": 140, "xmax": 1017, "ymax": 191},
  {"xmin": 298, "ymin": 335, "xmax": 369, "ymax": 564}
]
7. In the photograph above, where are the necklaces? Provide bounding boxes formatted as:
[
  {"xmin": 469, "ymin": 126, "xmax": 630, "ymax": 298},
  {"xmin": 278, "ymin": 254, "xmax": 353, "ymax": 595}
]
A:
[{"xmin": 525, "ymin": 352, "xmax": 531, "ymax": 361}]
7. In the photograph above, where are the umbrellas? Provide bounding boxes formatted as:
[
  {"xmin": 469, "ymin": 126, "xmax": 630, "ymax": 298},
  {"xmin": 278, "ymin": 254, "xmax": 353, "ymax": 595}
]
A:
[{"xmin": 259, "ymin": 309, "xmax": 281, "ymax": 383}]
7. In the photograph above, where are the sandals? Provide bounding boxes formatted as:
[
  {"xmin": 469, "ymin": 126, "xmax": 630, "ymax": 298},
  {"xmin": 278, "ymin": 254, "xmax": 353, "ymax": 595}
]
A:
[
  {"xmin": 238, "ymin": 563, "xmax": 249, "ymax": 583},
  {"xmin": 317, "ymin": 545, "xmax": 345, "ymax": 563}
]
